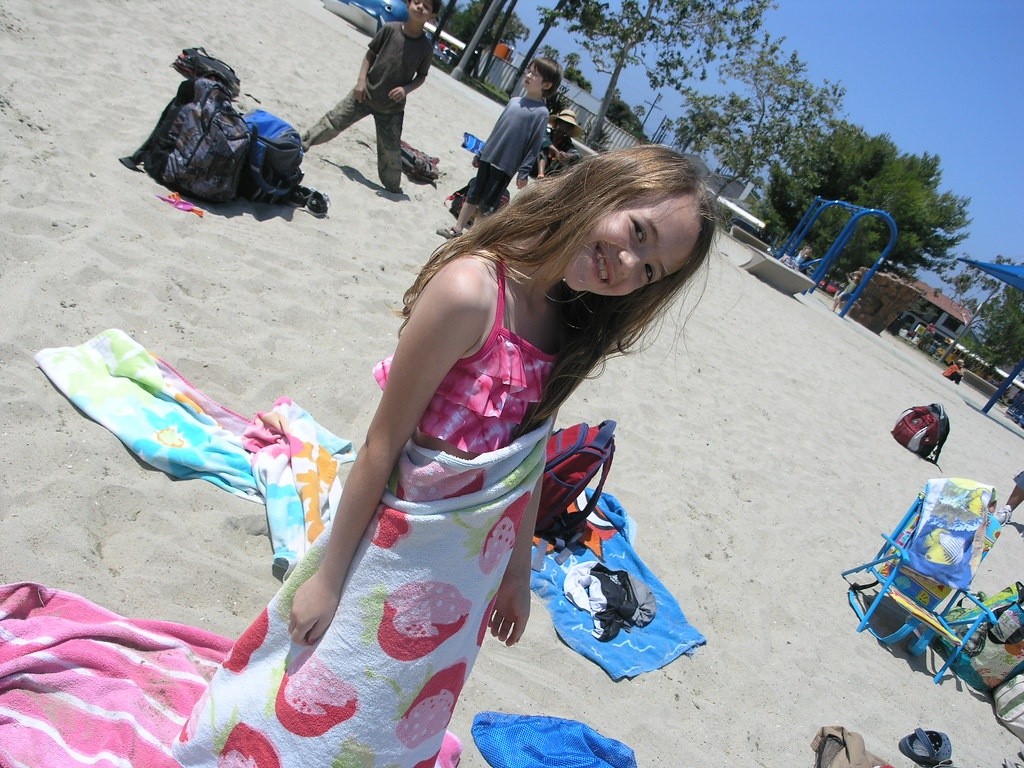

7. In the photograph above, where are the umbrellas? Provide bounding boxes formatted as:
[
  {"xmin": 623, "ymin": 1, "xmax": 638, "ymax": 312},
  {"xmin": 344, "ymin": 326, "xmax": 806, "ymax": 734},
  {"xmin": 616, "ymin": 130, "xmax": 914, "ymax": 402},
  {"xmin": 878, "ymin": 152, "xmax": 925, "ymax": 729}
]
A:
[{"xmin": 936, "ymin": 258, "xmax": 1024, "ymax": 414}]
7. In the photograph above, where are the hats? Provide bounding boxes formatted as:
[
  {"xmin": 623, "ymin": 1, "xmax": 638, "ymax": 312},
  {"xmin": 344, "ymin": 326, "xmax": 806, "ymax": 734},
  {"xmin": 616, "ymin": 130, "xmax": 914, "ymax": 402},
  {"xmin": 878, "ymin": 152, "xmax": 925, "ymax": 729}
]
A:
[
  {"xmin": 955, "ymin": 360, "xmax": 963, "ymax": 366},
  {"xmin": 548, "ymin": 109, "xmax": 584, "ymax": 137}
]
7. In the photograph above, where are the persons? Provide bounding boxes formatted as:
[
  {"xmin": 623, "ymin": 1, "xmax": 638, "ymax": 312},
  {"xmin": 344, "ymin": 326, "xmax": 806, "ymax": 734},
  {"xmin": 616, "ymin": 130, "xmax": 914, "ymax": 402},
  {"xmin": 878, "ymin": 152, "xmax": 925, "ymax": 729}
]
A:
[
  {"xmin": 942, "ymin": 359, "xmax": 965, "ymax": 384},
  {"xmin": 299, "ymin": 0, "xmax": 443, "ymax": 194},
  {"xmin": 830, "ymin": 271, "xmax": 862, "ymax": 312},
  {"xmin": 530, "ymin": 110, "xmax": 584, "ymax": 178},
  {"xmin": 994, "ymin": 471, "xmax": 1024, "ymax": 527},
  {"xmin": 779, "ymin": 246, "xmax": 813, "ymax": 274},
  {"xmin": 436, "ymin": 58, "xmax": 563, "ymax": 238},
  {"xmin": 170, "ymin": 145, "xmax": 712, "ymax": 768}
]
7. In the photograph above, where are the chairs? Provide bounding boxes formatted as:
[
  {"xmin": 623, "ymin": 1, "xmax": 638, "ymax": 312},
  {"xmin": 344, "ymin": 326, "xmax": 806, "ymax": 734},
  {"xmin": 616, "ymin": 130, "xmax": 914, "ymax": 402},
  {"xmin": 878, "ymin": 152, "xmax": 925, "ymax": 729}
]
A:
[{"xmin": 842, "ymin": 481, "xmax": 1003, "ymax": 684}]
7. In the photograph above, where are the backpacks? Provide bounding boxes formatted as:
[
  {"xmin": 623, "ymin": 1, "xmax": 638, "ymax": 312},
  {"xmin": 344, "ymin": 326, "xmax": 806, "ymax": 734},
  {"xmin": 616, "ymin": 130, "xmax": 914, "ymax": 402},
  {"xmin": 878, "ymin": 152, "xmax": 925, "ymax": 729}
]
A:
[
  {"xmin": 118, "ymin": 76, "xmax": 253, "ymax": 204},
  {"xmin": 891, "ymin": 402, "xmax": 950, "ymax": 464},
  {"xmin": 535, "ymin": 420, "xmax": 616, "ymax": 532},
  {"xmin": 170, "ymin": 46, "xmax": 242, "ymax": 98},
  {"xmin": 238, "ymin": 108, "xmax": 306, "ymax": 205}
]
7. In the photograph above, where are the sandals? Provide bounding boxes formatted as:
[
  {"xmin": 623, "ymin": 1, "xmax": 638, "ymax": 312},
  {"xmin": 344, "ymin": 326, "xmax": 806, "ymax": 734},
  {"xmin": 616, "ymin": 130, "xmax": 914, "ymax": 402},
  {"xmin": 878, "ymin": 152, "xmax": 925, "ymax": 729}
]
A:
[{"xmin": 899, "ymin": 727, "xmax": 952, "ymax": 765}]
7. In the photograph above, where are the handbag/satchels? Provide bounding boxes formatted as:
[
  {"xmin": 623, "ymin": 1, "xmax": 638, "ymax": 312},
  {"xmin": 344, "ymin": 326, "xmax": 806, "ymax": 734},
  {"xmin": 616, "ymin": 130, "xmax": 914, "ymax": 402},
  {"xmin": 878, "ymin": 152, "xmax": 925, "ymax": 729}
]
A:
[
  {"xmin": 444, "ymin": 176, "xmax": 510, "ymax": 229},
  {"xmin": 399, "ymin": 140, "xmax": 440, "ymax": 186}
]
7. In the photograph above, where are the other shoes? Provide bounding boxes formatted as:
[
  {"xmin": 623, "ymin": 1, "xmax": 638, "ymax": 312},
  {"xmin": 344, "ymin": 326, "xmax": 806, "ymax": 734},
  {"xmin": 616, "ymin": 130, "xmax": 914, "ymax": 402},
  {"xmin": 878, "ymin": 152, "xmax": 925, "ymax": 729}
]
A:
[
  {"xmin": 993, "ymin": 504, "xmax": 1013, "ymax": 527},
  {"xmin": 304, "ymin": 191, "xmax": 331, "ymax": 217},
  {"xmin": 284, "ymin": 187, "xmax": 315, "ymax": 209},
  {"xmin": 436, "ymin": 227, "xmax": 463, "ymax": 239}
]
